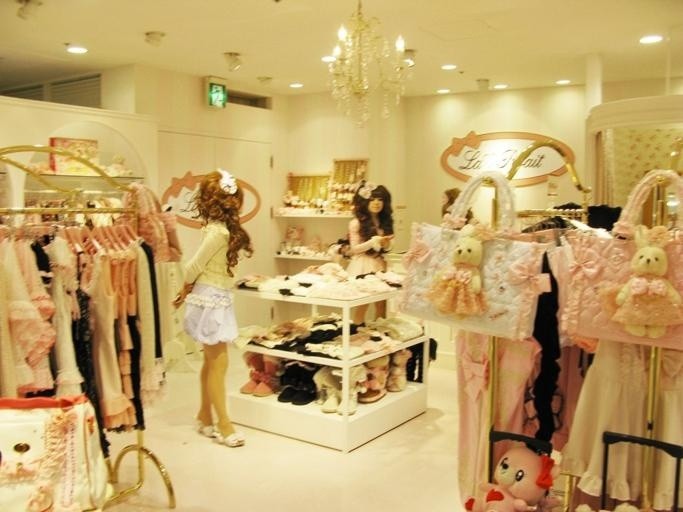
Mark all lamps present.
[227,54,242,72]
[399,48,416,69]
[17,1,38,21]
[322,0,408,127]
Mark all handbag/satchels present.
[557,168,683,351]
[0,397,108,512]
[397,172,550,341]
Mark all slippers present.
[194,419,217,438]
[214,424,245,447]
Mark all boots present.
[312,349,412,414]
[240,352,319,405]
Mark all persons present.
[172,169,254,448]
[441,188,474,224]
[348,179,394,324]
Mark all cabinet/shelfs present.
[270,206,354,261]
[225,287,429,453]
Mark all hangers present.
[1,208,177,266]
[518,206,587,234]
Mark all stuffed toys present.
[425,224,486,320]
[465,444,554,512]
[610,225,683,339]
